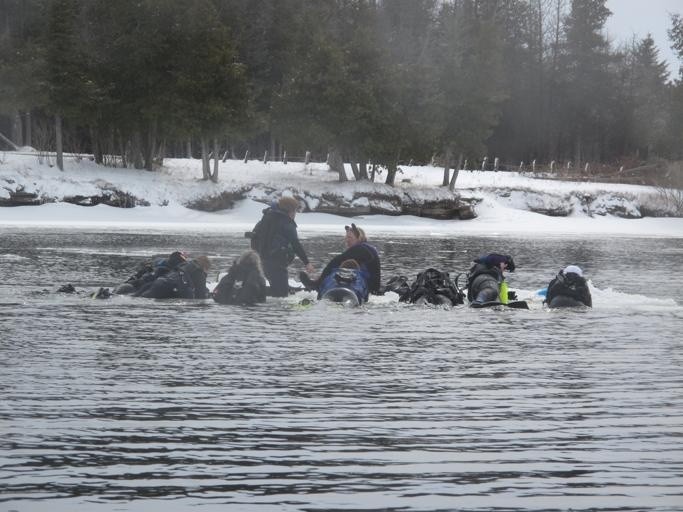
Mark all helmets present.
[564,266,582,276]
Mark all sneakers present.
[300,271,312,290]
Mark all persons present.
[297,222,380,294]
[317,259,370,310]
[545,265,591,308]
[468,251,515,306]
[132,255,211,300]
[116,250,187,297]
[251,195,312,298]
[211,249,265,305]
